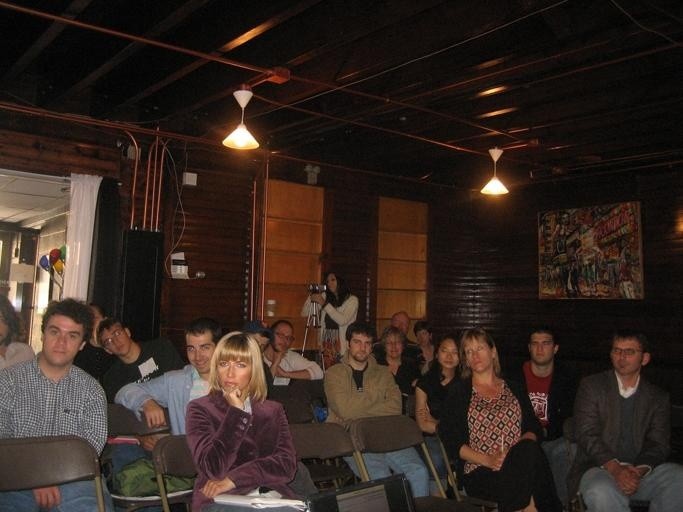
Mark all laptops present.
[311,473,414,512]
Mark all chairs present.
[562,417,585,512]
[1,435,105,511]
[104,403,195,512]
[268,379,498,511]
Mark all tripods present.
[300,300,322,361]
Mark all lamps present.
[479,147,513,197]
[221,86,263,151]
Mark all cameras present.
[308,284,327,291]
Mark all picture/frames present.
[533,202,647,304]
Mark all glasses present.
[274,331,295,342]
[102,327,125,348]
[611,347,644,355]
